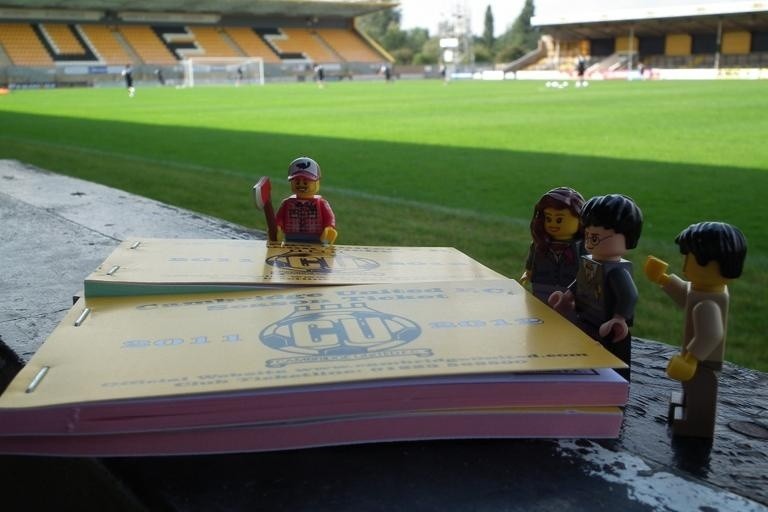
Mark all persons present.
[121,60,136,98]
[377,64,390,82]
[439,59,447,84]
[573,46,588,88]
[312,62,325,88]
[252,157,337,246]
[547,194,645,390]
[518,187,585,323]
[643,221,748,436]
[236,64,245,86]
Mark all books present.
[0,407,625,461]
[1,298,630,433]
[84,236,509,299]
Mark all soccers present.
[544,80,568,88]
[575,82,588,87]
